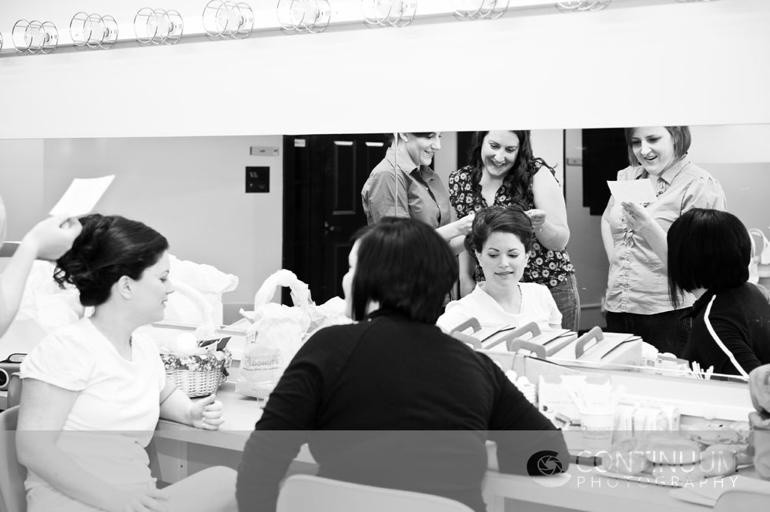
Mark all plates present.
[609,422,738,476]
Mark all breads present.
[602,430,752,483]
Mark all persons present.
[15,215,237,512]
[600,127,770,380]
[0,196,82,340]
[237,218,568,511]
[362,130,581,335]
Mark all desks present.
[0,326,769,511]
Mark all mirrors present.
[0,120,768,410]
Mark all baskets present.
[165,368,223,398]
